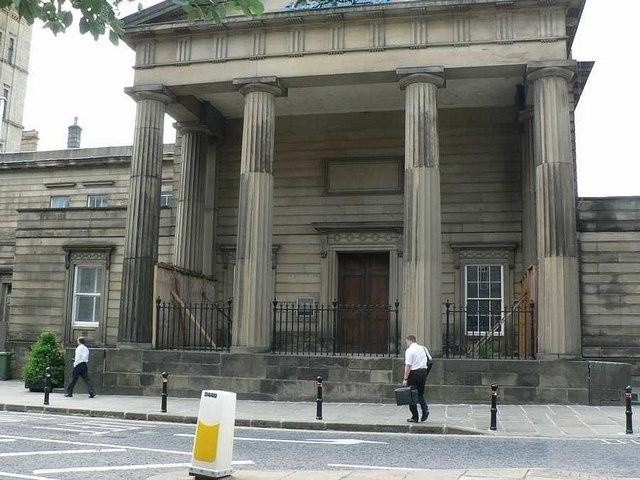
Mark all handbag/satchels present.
[394,383,420,405]
[426,355,432,376]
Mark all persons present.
[64,337,97,399]
[403,335,432,422]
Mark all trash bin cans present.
[0,351,15,380]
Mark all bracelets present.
[404,378,407,380]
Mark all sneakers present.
[405,410,430,424]
[64,391,97,399]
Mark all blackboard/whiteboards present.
[297,297,315,317]
